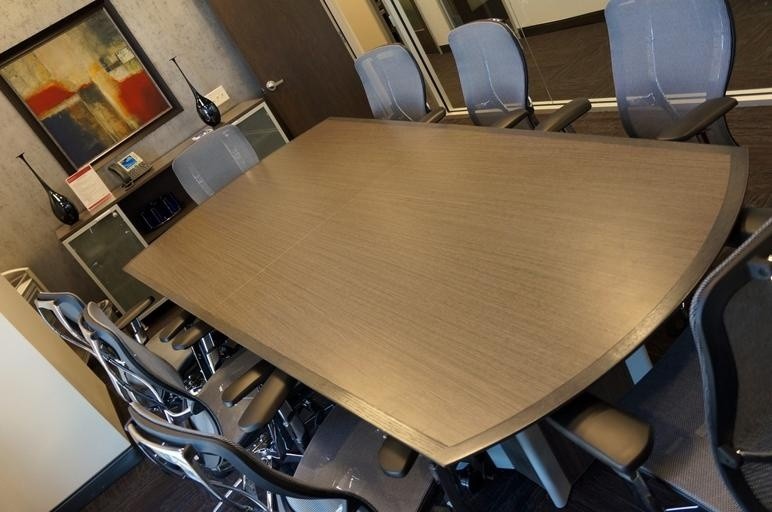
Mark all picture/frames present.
[0,0,184,177]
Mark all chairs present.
[171,124,261,206]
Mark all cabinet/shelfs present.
[54,95,292,322]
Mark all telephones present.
[105,150,153,187]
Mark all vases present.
[169,55,221,127]
[15,152,80,226]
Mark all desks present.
[122,114,750,510]
